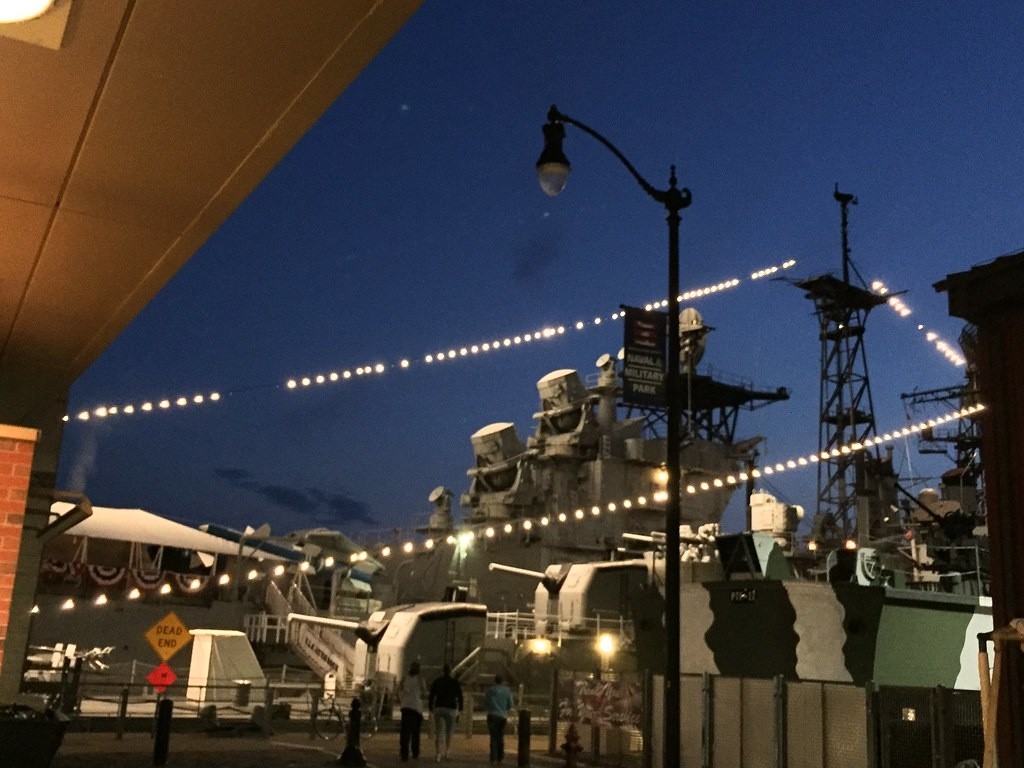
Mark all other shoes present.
[401,753,408,761]
[411,753,419,758]
[496,761,502,767]
[489,760,494,765]
[445,751,449,760]
[436,754,440,762]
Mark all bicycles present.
[313,693,377,742]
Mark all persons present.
[428,664,463,764]
[484,675,513,763]
[395,662,430,760]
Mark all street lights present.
[535,106,693,767]
[530,638,558,757]
[809,541,817,581]
